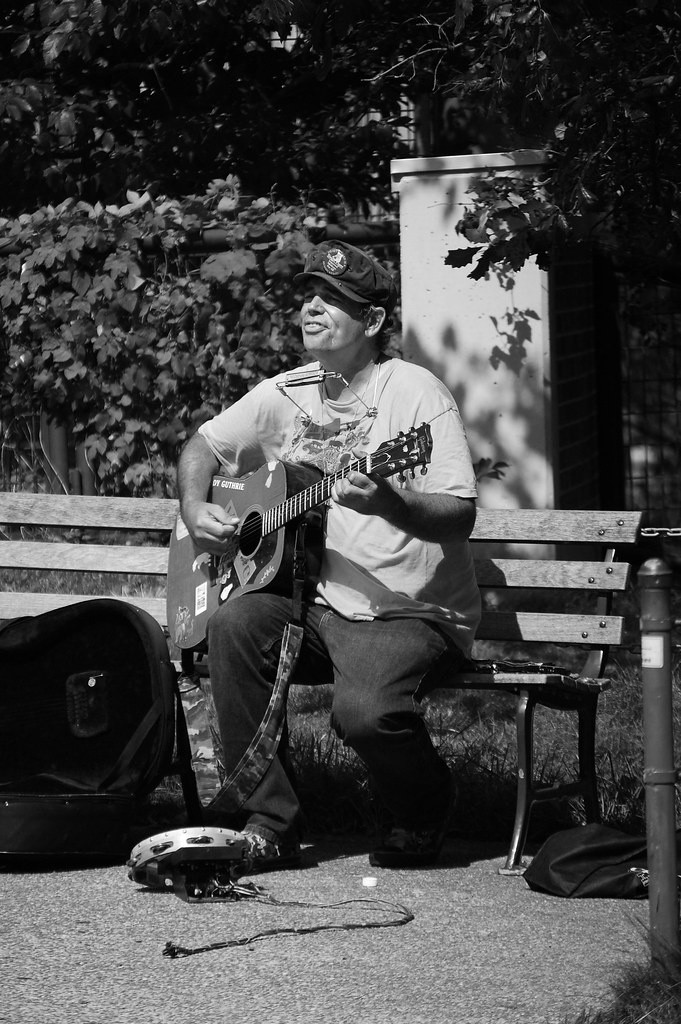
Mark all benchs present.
[0,491,642,874]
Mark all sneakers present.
[368,780,464,870]
[232,831,303,875]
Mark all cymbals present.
[128,826,253,891]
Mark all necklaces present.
[320,355,374,510]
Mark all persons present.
[172,246,486,869]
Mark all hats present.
[292,239,397,318]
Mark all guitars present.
[161,421,434,652]
